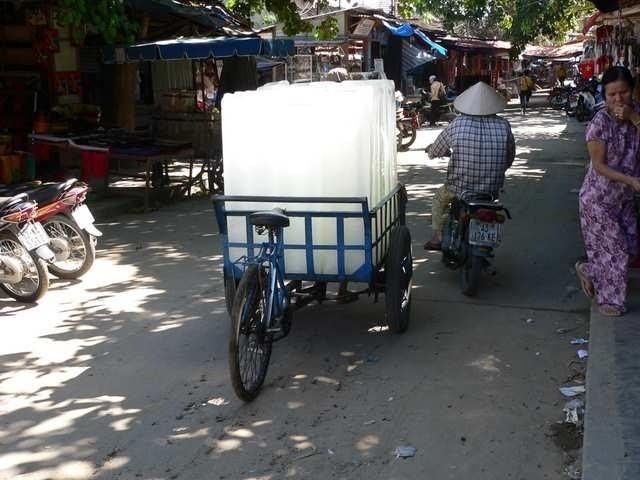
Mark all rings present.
[615,113,619,117]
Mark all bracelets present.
[632,120,640,128]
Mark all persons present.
[423,75,448,127]
[427,81,515,251]
[576,65,640,317]
[518,61,566,106]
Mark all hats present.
[454,80,507,115]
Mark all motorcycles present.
[0,174,104,280]
[423,140,509,298]
[0,192,55,303]
[396,84,464,153]
[547,75,603,122]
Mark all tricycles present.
[207,176,414,405]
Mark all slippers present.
[599,306,623,316]
[423,241,441,250]
[574,260,594,298]
